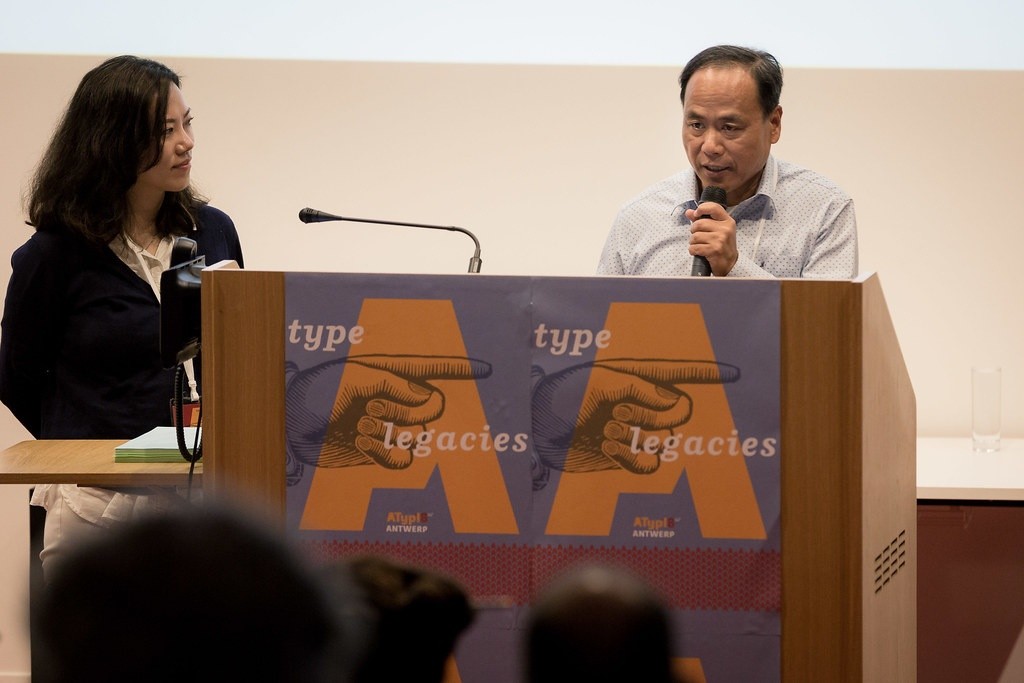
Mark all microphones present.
[300,208,481,273]
[690,184,727,277]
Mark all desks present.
[0,439,203,683]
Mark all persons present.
[596,45,860,281]
[26,488,695,683]
[0,55,246,581]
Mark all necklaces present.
[145,236,155,250]
